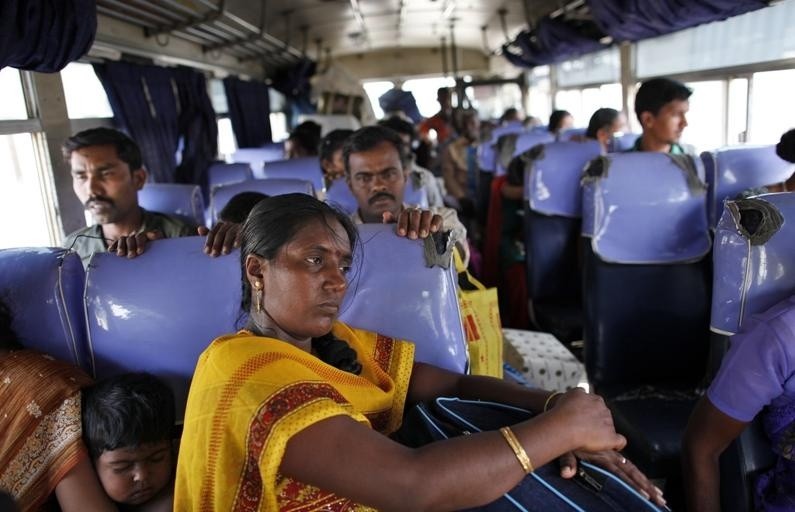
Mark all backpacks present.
[397,394,673,512]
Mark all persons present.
[548,80,694,153]
[736,128,795,200]
[682,294,795,511]
[62,111,469,274]
[2,192,665,511]
[378,80,547,237]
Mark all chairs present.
[474,122,794,509]
[1,142,473,435]
